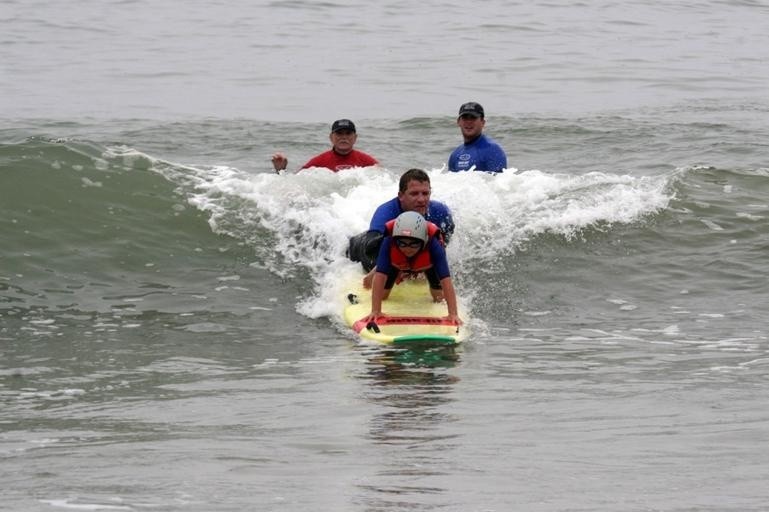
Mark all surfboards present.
[343,256,464,344]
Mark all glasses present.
[396,241,419,249]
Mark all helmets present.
[392,210,429,251]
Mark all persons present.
[363,209,464,326]
[447,101,506,174]
[367,170,456,248]
[269,119,380,174]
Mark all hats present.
[457,101,484,120]
[332,119,356,133]
